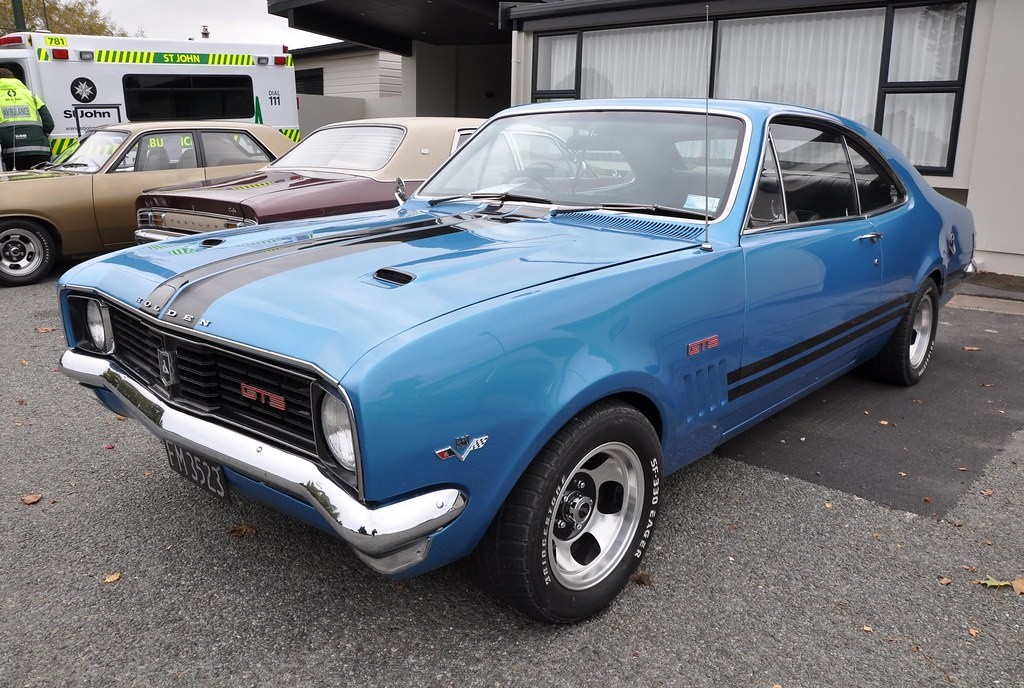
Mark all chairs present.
[144,147,169,171]
[175,149,197,169]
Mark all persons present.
[0,68,57,171]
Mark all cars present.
[0,121,301,289]
[134,116,627,246]
[57,98,978,626]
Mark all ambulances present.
[0,30,302,172]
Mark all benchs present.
[667,165,883,224]
